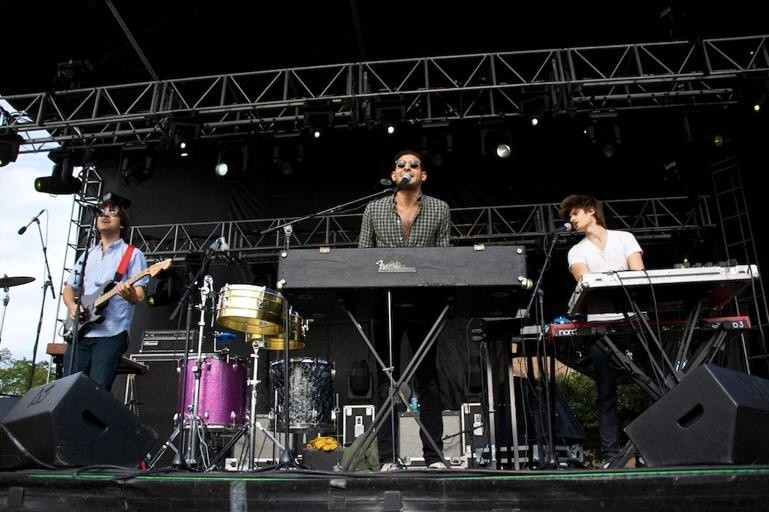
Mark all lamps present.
[0,70,768,194]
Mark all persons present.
[358,150,451,471]
[559,195,645,468]
[62,198,150,392]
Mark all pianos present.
[566,262,760,316]
[546,315,760,343]
[276,245,528,321]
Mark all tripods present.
[146,296,215,476]
[201,339,285,474]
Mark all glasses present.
[395,159,422,168]
[96,208,123,219]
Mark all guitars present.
[63,259,172,342]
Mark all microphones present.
[18,208,46,236]
[395,172,411,195]
[209,236,225,252]
[87,203,105,217]
[546,221,572,239]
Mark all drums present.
[173,352,247,435]
[244,307,306,350]
[216,284,282,336]
[270,358,336,431]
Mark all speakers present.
[622,362,768,467]
[0,370,158,472]
